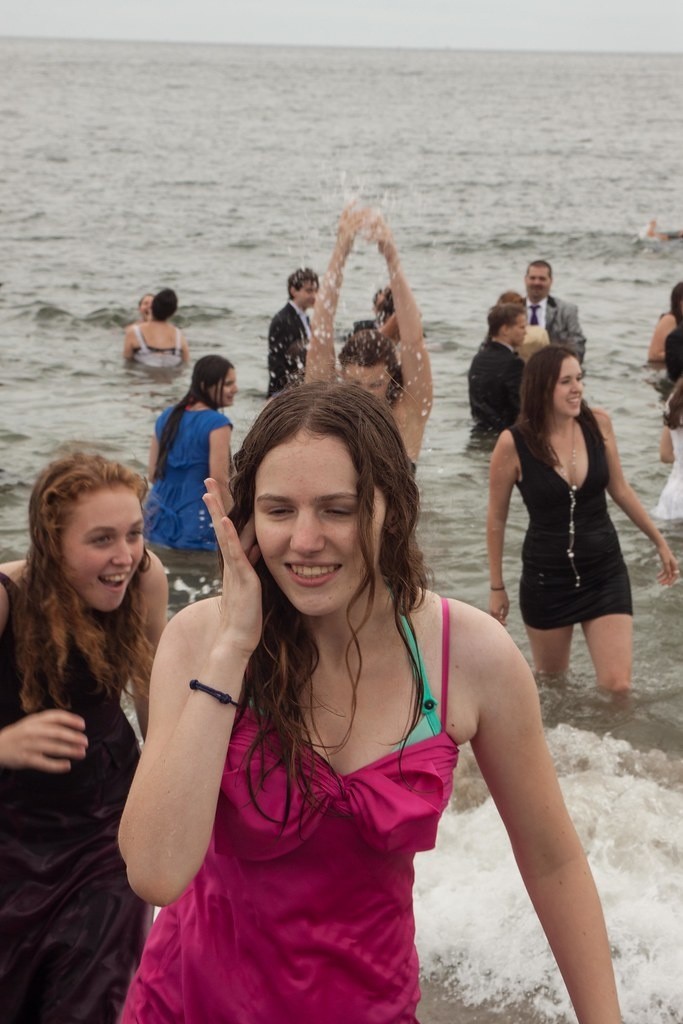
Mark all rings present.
[671,569,680,574]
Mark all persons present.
[117,382,625,1024]
[266,199,434,476]
[123,289,189,367]
[142,354,238,550]
[647,283,683,519]
[466,260,588,451]
[487,345,680,695]
[0,450,169,1024]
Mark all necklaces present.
[547,417,581,589]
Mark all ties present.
[305,316,312,331]
[529,303,540,323]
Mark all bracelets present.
[189,679,240,708]
[490,585,505,591]
